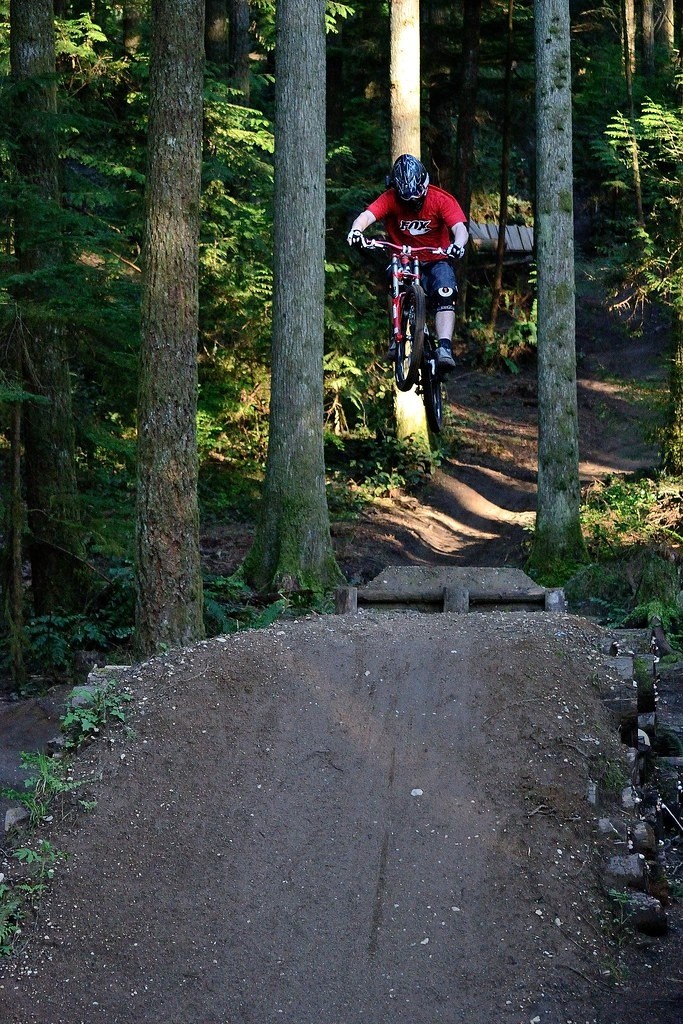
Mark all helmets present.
[392,153,427,203]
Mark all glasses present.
[400,172,429,200]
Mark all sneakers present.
[435,346,456,368]
[387,341,396,357]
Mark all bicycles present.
[361,238,447,434]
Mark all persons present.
[347,154,469,373]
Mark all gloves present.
[347,229,363,248]
[445,243,465,259]
